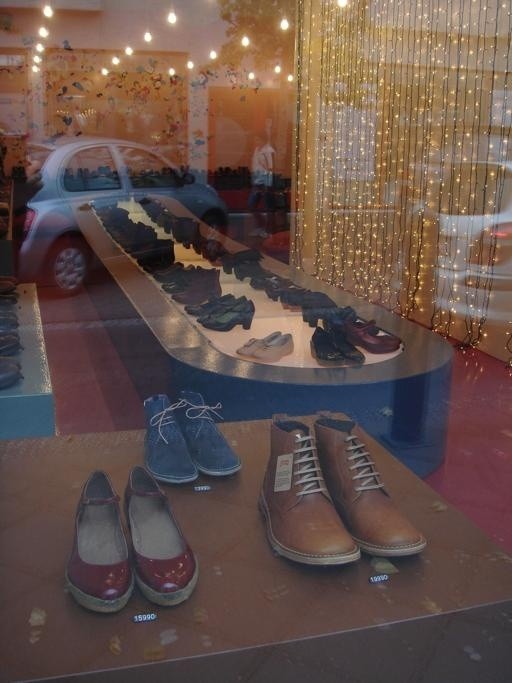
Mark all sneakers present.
[248,228,272,239]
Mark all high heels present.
[91,192,402,367]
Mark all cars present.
[389,161,512,323]
[15,133,231,298]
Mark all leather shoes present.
[65,464,199,614]
[257,412,428,565]
[64,165,252,191]
[143,390,242,483]
[0,275,21,392]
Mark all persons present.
[244,125,278,240]
